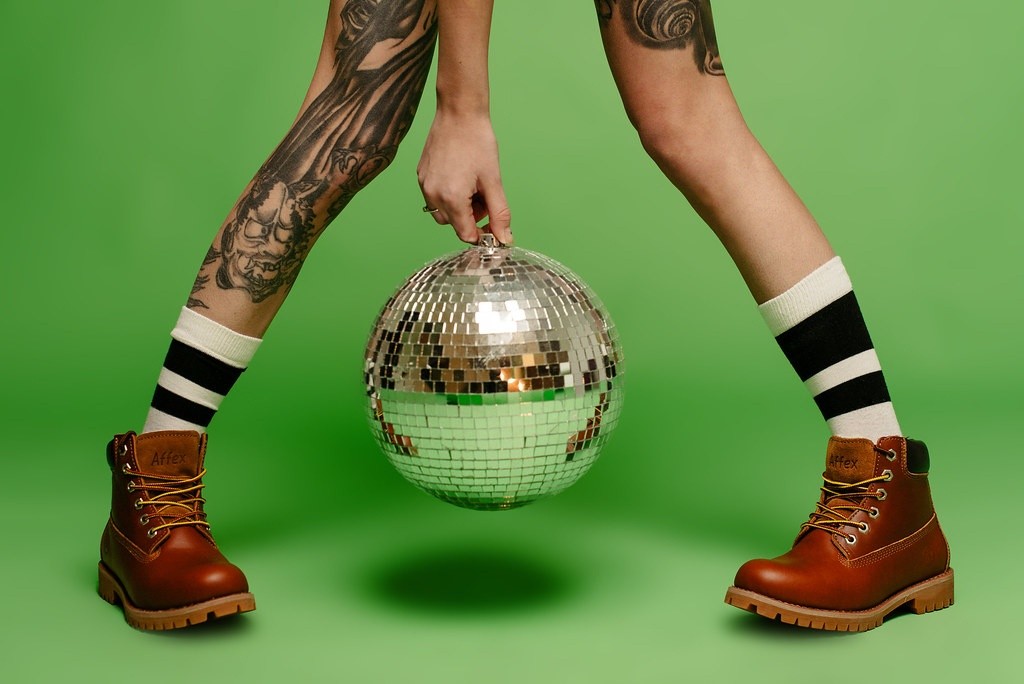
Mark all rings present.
[422,206,438,215]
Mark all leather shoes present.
[96,431,256,628]
[724,434,955,632]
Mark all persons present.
[94,0,955,634]
